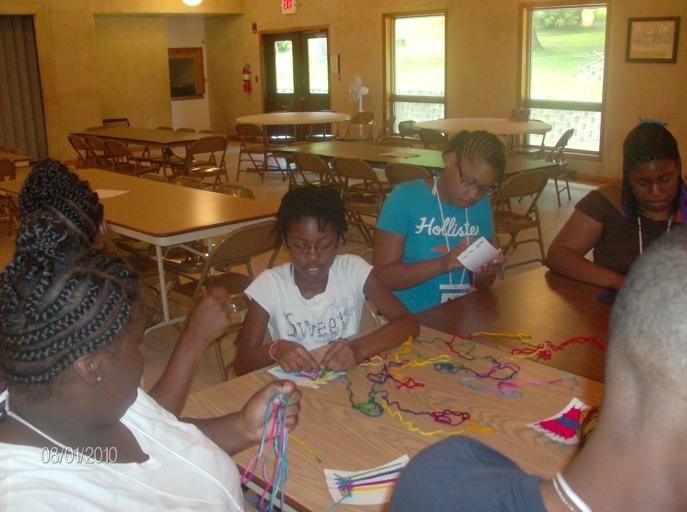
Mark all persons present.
[1,158,303,512]
[546,123,687,291]
[234,190,420,372]
[387,226,687,511]
[371,130,506,315]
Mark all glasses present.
[283,234,346,256]
[457,158,501,197]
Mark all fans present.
[345,74,370,113]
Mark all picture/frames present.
[626,15,680,64]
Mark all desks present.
[179,267,617,512]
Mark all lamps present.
[182,0,202,6]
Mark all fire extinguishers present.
[243,64,251,92]
